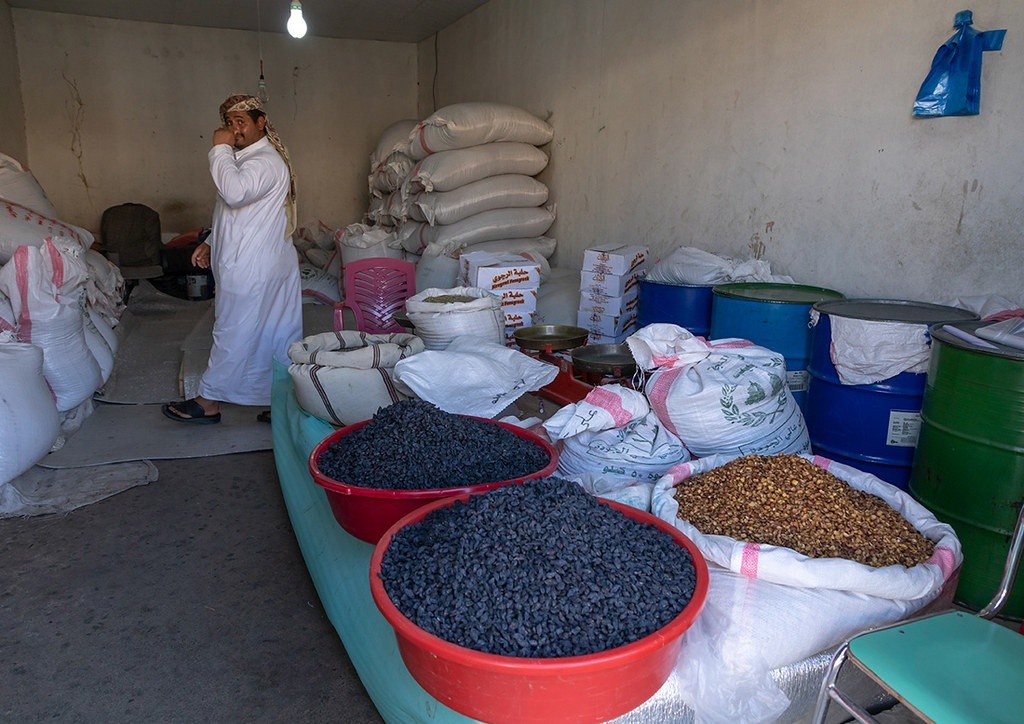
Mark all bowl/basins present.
[369,490,710,723]
[309,415,559,545]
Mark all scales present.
[513,324,637,407]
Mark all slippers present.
[258,410,273,423]
[162,398,222,423]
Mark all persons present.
[162,95,302,424]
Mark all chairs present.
[100,204,176,297]
[813,508,1024,724]
[332,257,417,331]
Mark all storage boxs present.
[455,250,542,350]
[577,243,652,346]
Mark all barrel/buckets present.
[632,273,712,341]
[801,297,981,490]
[710,282,849,409]
[906,319,1024,623]
